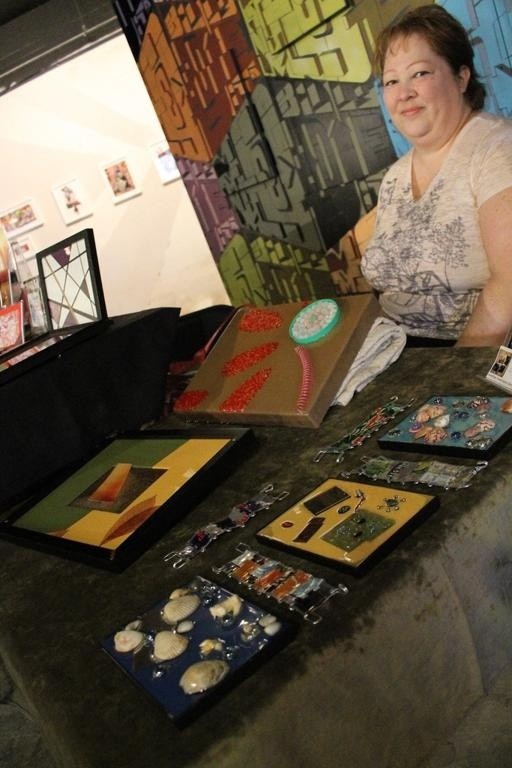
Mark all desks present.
[0,345,511,767]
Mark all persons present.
[358,2,512,350]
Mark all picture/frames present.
[0,426,260,573]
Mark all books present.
[0,300,25,348]
